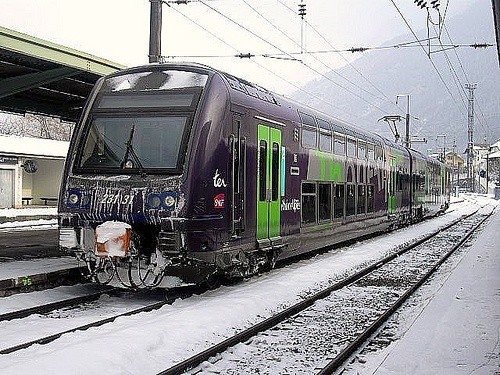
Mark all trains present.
[56,61,455,292]
